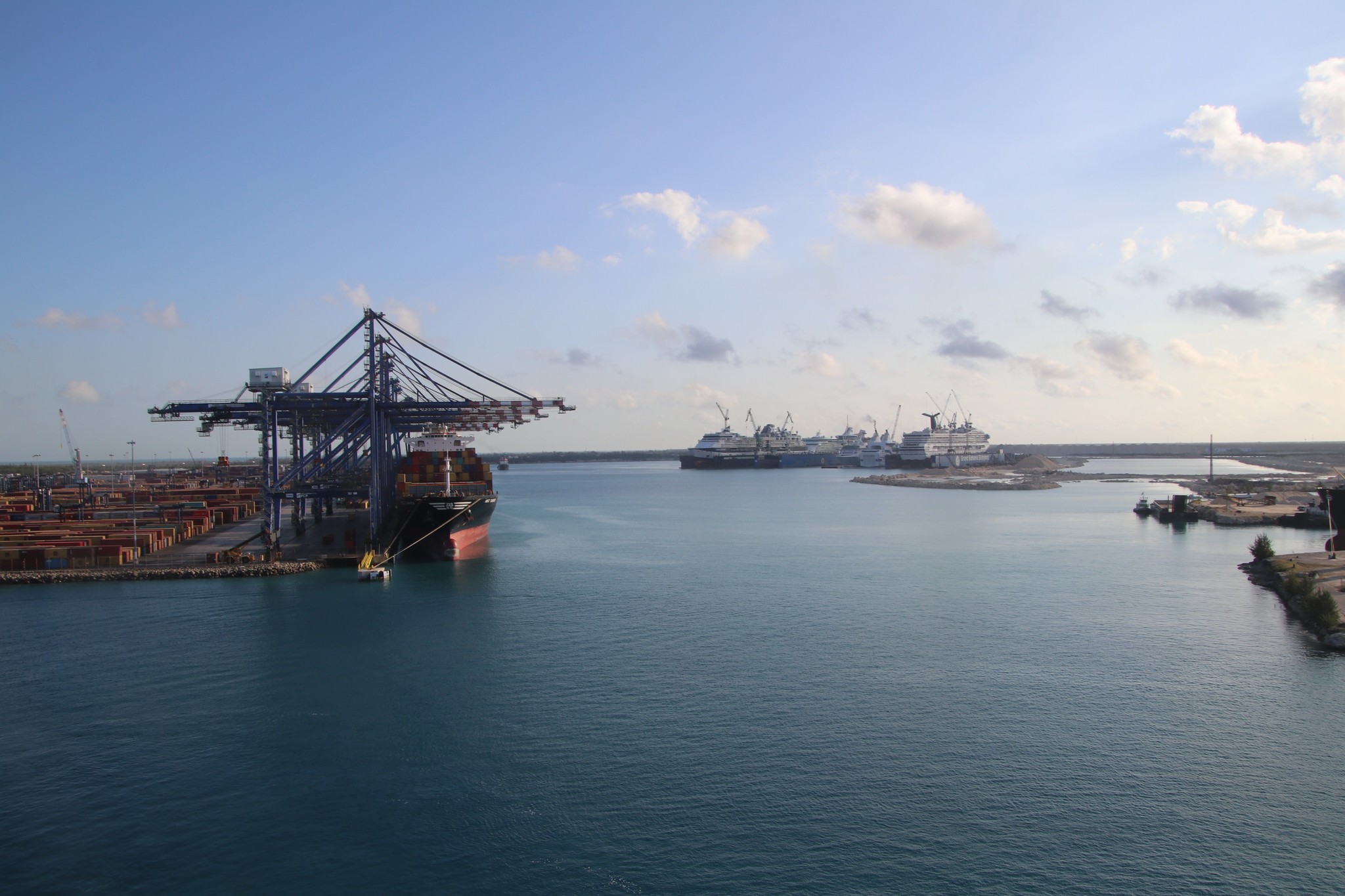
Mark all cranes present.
[148,307,580,576]
[59,408,88,485]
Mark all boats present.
[677,400,862,470]
[897,386,990,465]
[835,414,866,467]
[393,421,499,556]
[859,405,906,467]
[498,454,509,470]
[357,566,392,581]
[1133,491,1152,513]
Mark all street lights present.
[168,451,172,483]
[85,455,89,479]
[127,440,139,565]
[109,454,114,498]
[32,454,41,489]
[200,451,204,477]
